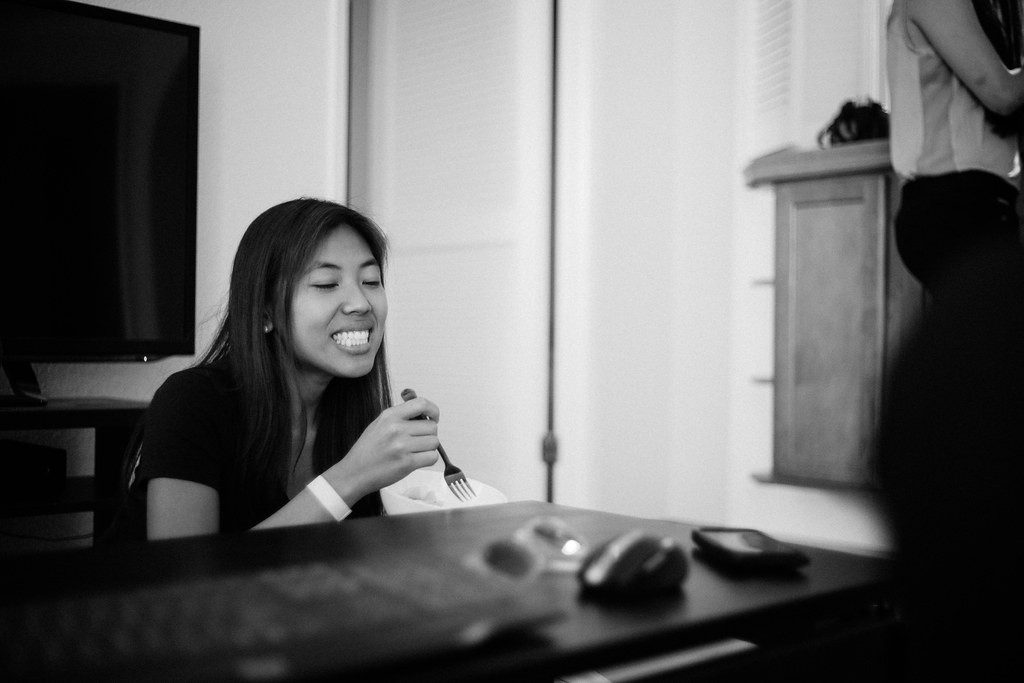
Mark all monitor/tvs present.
[0,0,200,403]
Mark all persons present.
[884,0,1023,297]
[123,200,439,541]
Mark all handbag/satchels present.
[818,101,889,145]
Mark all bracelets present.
[306,476,353,522]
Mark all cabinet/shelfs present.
[745,142,944,489]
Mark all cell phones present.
[691,527,806,570]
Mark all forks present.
[400,388,477,502]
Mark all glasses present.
[469,516,588,587]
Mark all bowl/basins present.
[380,469,507,516]
